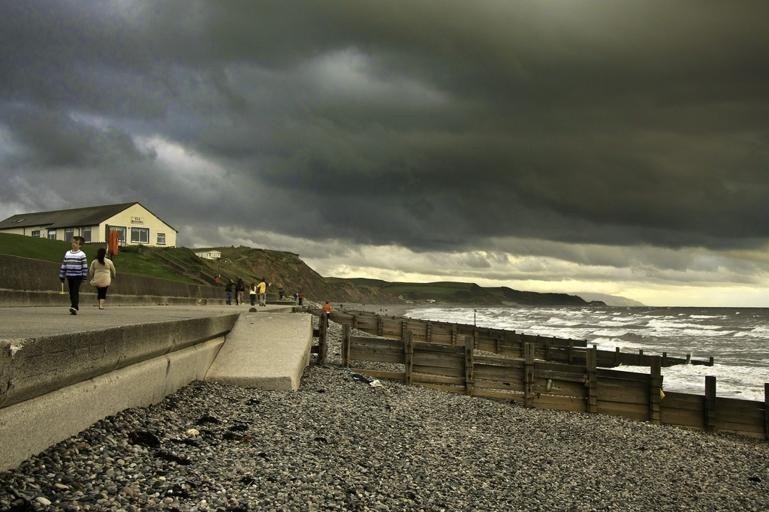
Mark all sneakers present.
[70,308,78,314]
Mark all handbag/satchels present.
[90,280,95,287]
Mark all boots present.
[99,299,105,310]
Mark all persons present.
[59,237,88,315]
[89,248,116,310]
[279,287,304,305]
[225,277,272,307]
[322,301,333,330]
